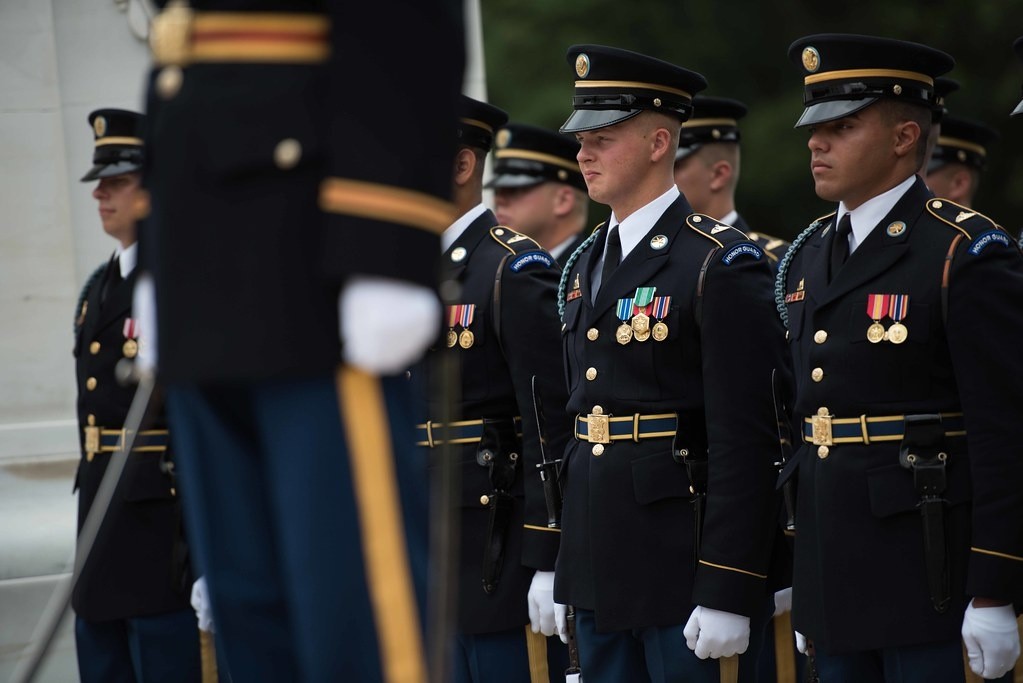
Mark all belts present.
[570,413,675,444]
[801,412,968,446]
[413,417,524,448]
[139,3,331,64]
[81,424,172,453]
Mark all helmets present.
[459,95,508,152]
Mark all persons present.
[774,34,1023,683]
[927,116,1000,225]
[408,90,568,683]
[672,94,794,615]
[133,0,468,682]
[553,45,775,683]
[480,119,589,269]
[72,108,213,683]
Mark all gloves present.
[528,569,570,644]
[961,596,1019,682]
[795,632,811,661]
[337,274,443,376]
[684,604,751,659]
[773,588,794,618]
[189,575,215,635]
[130,273,159,376]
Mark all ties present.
[602,224,622,287]
[828,215,852,285]
[105,256,121,298]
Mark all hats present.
[676,96,747,163]
[484,122,588,194]
[558,43,709,133]
[927,118,993,173]
[787,35,955,130]
[80,109,151,181]
[929,79,960,121]
